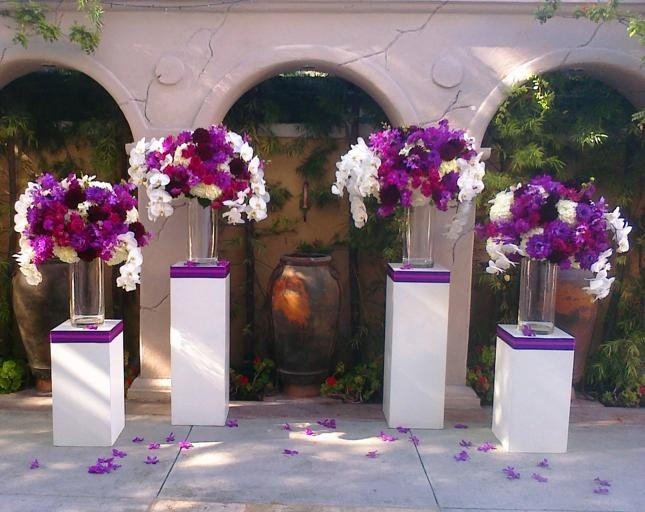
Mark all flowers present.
[15,171,152,292]
[485,175,632,303]
[126,122,271,226]
[329,115,485,244]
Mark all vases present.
[519,258,556,334]
[269,252,354,385]
[557,283,600,387]
[10,260,72,379]
[68,254,104,329]
